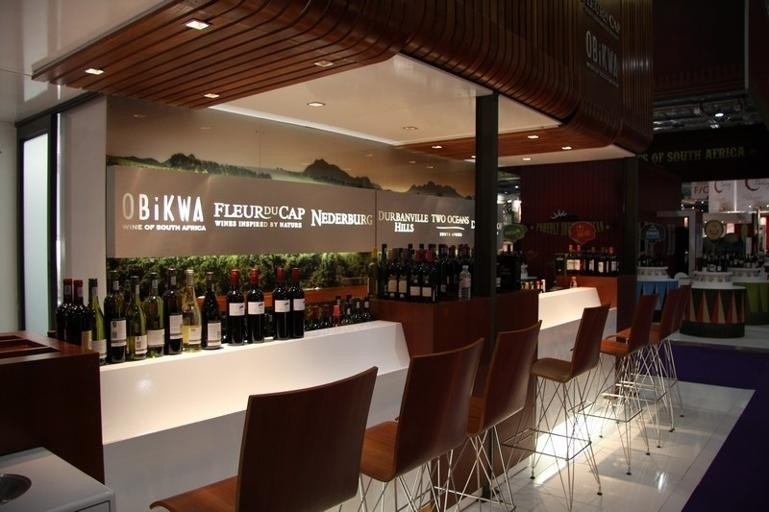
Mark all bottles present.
[308,294,374,333]
[520,278,554,294]
[571,276,577,290]
[54,266,305,365]
[368,242,527,302]
[637,249,765,274]
[566,243,619,276]
